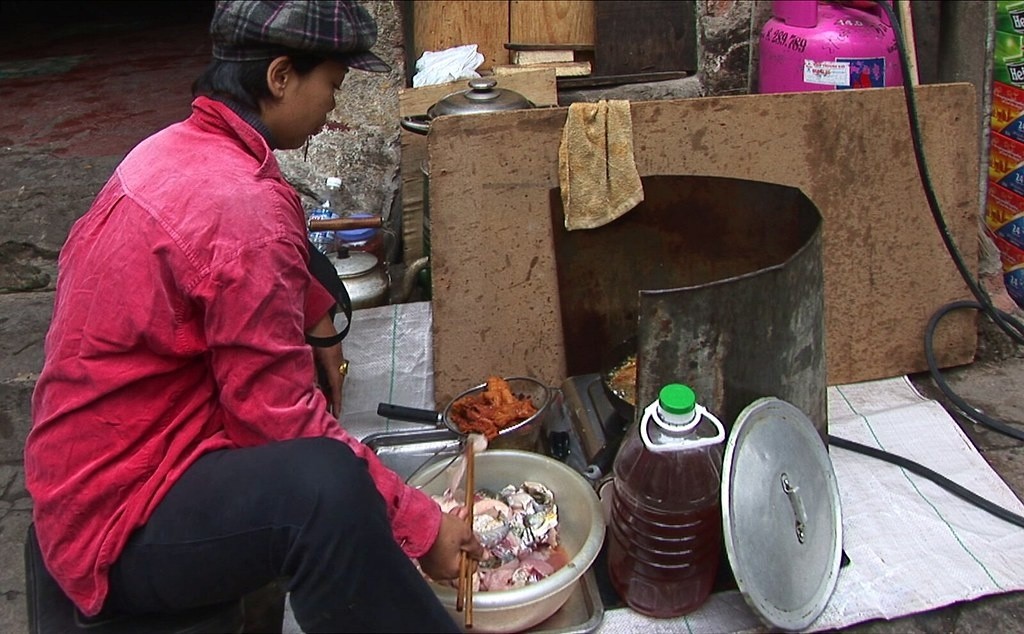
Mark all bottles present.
[307,176,345,255]
[608,382,727,620]
[336,212,385,267]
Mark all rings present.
[338,359,350,375]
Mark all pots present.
[603,330,639,423]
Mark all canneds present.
[336,214,384,267]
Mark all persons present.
[25,0,486,634]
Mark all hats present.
[210,0,392,73]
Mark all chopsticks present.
[456,438,474,628]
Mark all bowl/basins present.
[399,449,607,634]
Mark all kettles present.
[306,215,430,313]
